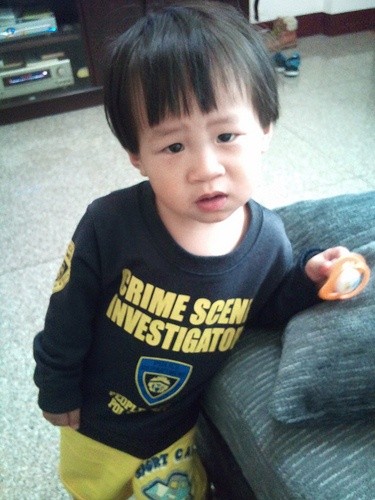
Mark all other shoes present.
[262,15,298,52]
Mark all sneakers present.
[270,52,301,76]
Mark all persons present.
[30,1,361,500]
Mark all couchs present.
[189,192,372,500]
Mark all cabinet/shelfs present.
[0,0,257,122]
